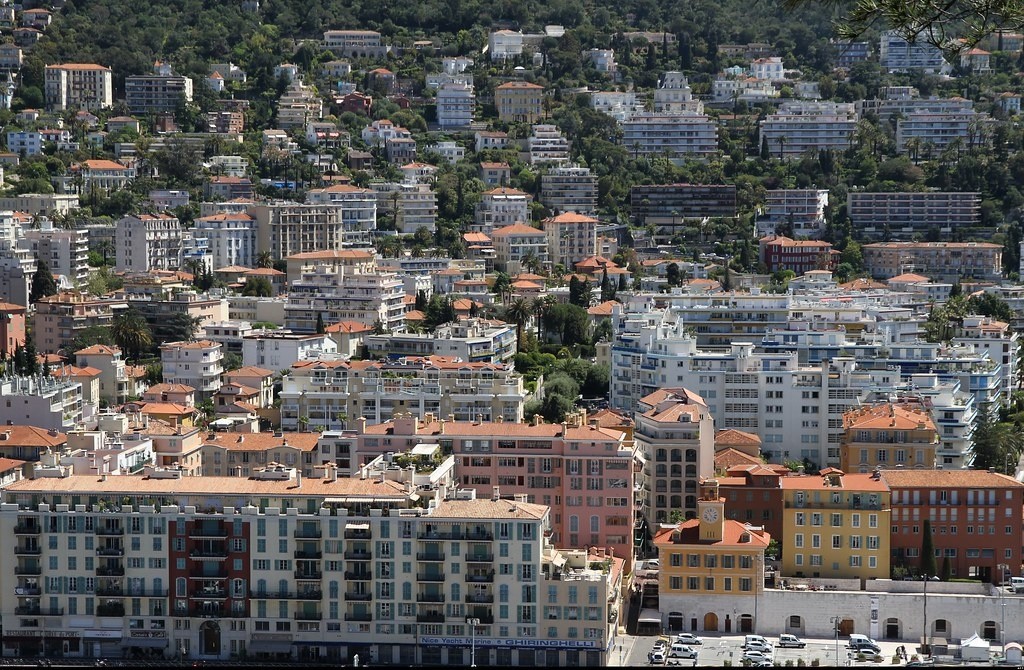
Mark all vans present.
[1008,577,1024,590]
[745,635,772,646]
[848,633,885,662]
[779,633,806,649]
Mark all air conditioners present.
[186,235,190,239]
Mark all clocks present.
[702,507,719,524]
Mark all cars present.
[752,662,774,667]
[742,651,773,662]
[745,642,771,652]
[649,635,698,665]
[641,560,659,570]
[677,633,703,646]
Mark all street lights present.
[922,574,941,653]
